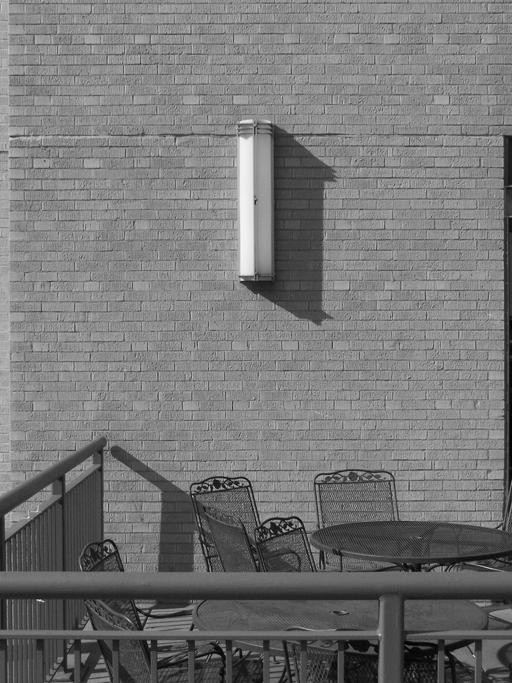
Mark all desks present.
[311,517,512,572]
[194,597,490,681]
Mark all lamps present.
[236,117,279,283]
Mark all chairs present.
[77,538,226,680]
